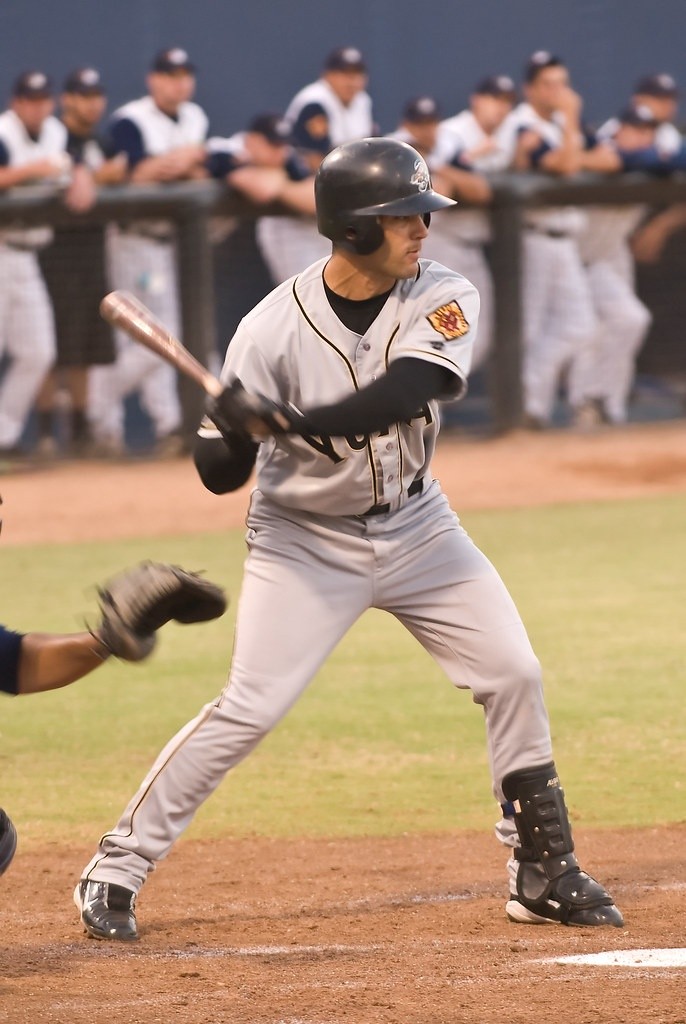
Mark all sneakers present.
[73,878,139,941]
[505,870,626,928]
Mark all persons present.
[206,114,316,246]
[72,137,625,940]
[437,76,518,373]
[581,72,686,423]
[36,67,128,459]
[0,70,96,464]
[390,97,489,202]
[493,49,603,428]
[84,48,251,459]
[257,44,374,282]
[0,489,228,879]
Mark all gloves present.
[205,377,296,447]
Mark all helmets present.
[526,51,561,80]
[17,70,53,99]
[64,68,103,96]
[153,47,194,75]
[313,138,458,256]
[470,73,516,100]
[406,95,438,121]
[325,46,364,71]
[636,73,678,99]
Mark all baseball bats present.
[99,287,268,433]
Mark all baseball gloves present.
[96,560,226,661]
